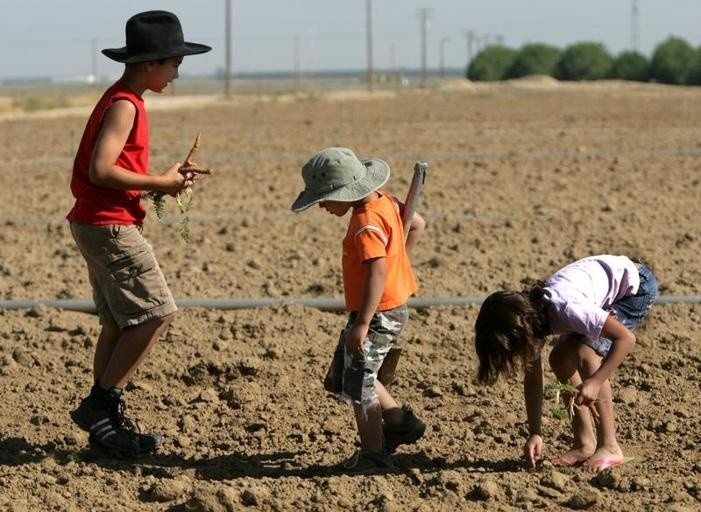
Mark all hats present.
[101,11,212,64]
[291,147,390,212]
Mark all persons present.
[66,10,211,459]
[291,147,427,473]
[475,253,658,475]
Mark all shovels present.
[324,161,426,393]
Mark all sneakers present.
[326,404,426,475]
[71,378,162,460]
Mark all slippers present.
[552,457,634,472]
